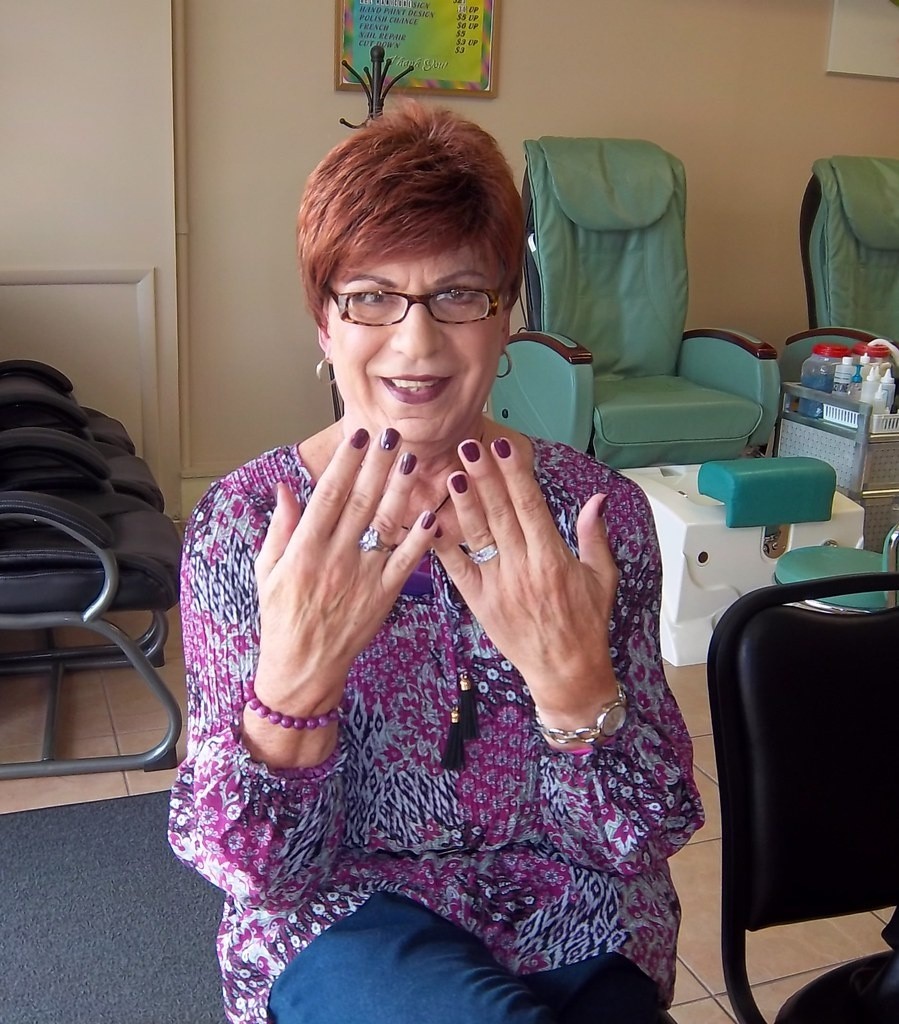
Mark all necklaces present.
[398,433,485,530]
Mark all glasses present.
[326,279,505,327]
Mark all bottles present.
[797,343,851,417]
[881,369,895,414]
[875,366,881,383]
[853,342,896,378]
[873,384,886,413]
[862,367,879,403]
[859,353,870,367]
[832,357,854,397]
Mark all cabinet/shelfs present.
[780,381,899,555]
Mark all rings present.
[469,544,498,563]
[358,526,395,552]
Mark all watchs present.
[535,682,627,743]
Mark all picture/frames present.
[334,0,503,99]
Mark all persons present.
[168,106,706,1024]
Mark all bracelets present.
[243,676,338,730]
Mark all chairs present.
[777,152,899,404]
[705,569,899,1023]
[480,136,782,468]
[0,357,183,773]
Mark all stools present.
[776,520,899,615]
